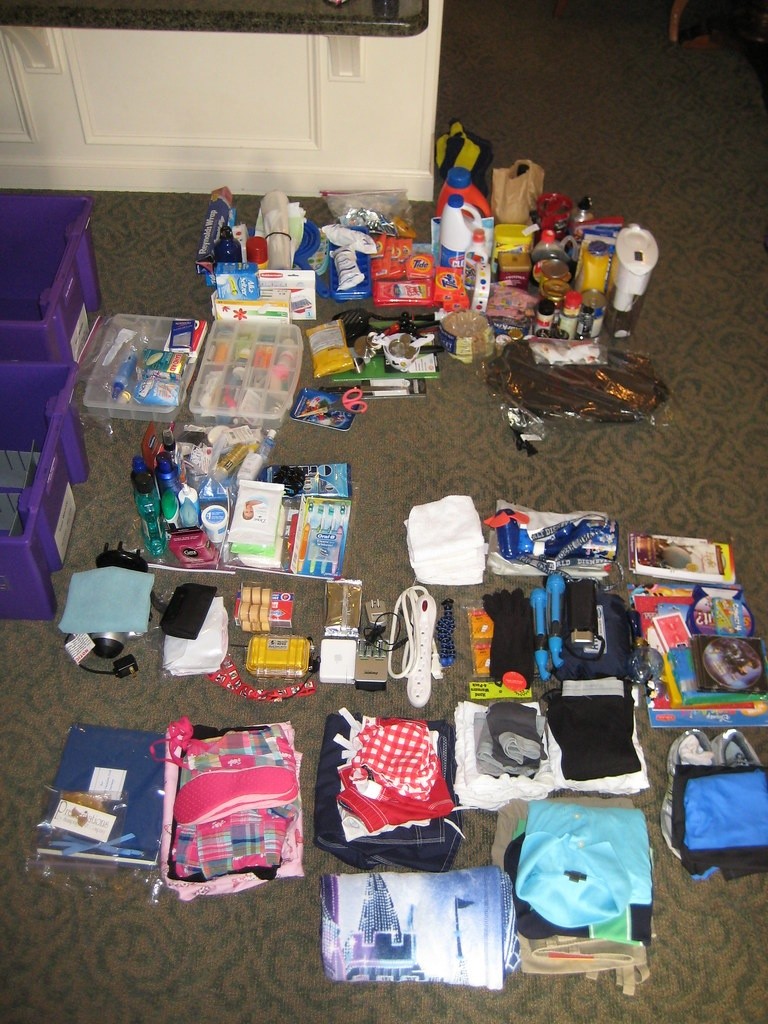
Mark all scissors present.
[293,387,368,418]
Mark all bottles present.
[215,226,242,263]
[535,229,578,263]
[441,195,480,267]
[437,167,491,217]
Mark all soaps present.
[371,236,471,311]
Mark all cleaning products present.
[436,166,492,292]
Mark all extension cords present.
[405,594,437,708]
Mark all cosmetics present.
[126,418,287,571]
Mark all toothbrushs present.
[297,500,346,574]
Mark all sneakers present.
[660,729,763,860]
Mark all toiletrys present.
[130,419,201,558]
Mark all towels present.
[402,493,486,586]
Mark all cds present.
[693,596,762,689]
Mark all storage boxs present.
[439,312,495,364]
[214,289,293,325]
[0,194,102,365]
[245,633,310,678]
[234,591,295,629]
[82,313,197,423]
[215,262,260,300]
[490,224,533,273]
[497,251,532,290]
[189,319,304,429]
[0,362,90,621]
[168,526,220,568]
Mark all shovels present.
[331,307,436,341]
